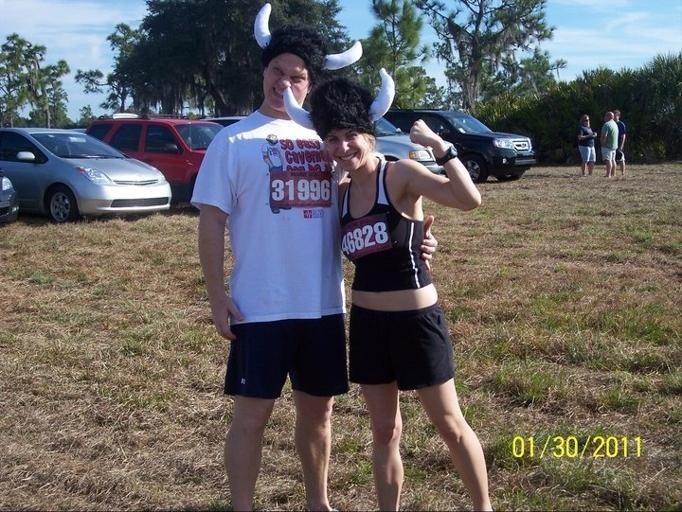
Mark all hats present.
[261,23,329,86]
[310,78,376,142]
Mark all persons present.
[284,69,491,511]
[191,24,439,511]
[578,110,626,178]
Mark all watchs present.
[436,145,458,167]
